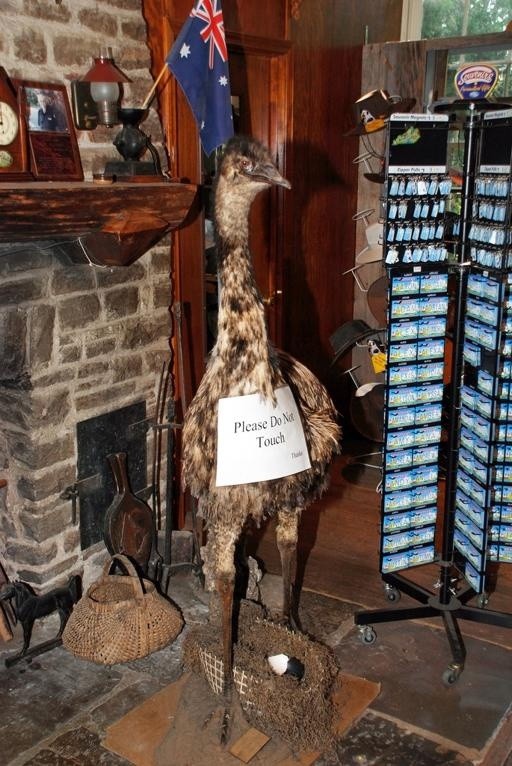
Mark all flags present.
[163,0,237,159]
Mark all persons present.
[35,93,63,130]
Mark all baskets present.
[61,554,186,666]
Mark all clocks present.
[0,66,35,182]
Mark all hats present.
[328,319,387,368]
[341,88,416,138]
[355,221,384,264]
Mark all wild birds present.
[179,135,345,752]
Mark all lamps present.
[71,46,128,130]
